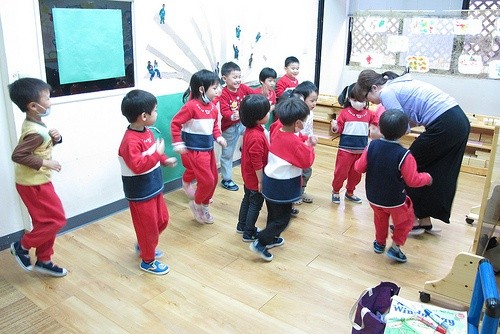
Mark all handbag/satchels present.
[349,280,396,334]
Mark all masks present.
[350,99,366,111]
[34,102,51,117]
[295,120,305,132]
[202,87,210,103]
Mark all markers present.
[424,308,449,329]
[418,315,446,334]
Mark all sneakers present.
[332,193,340,204]
[291,207,300,217]
[242,229,263,242]
[140,259,169,275]
[190,199,205,223]
[34,260,67,277]
[373,239,386,253]
[11,240,33,272]
[202,206,214,224]
[345,193,362,203]
[221,179,239,191]
[265,237,284,249]
[249,239,273,261]
[136,244,163,257]
[183,182,195,197]
[386,245,408,262]
[236,226,259,234]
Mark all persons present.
[236,94,270,243]
[249,98,314,262]
[353,69,471,235]
[9,77,68,277]
[253,67,277,112]
[274,56,300,104]
[220,62,267,191]
[117,90,179,275]
[273,80,319,217]
[354,109,433,262]
[171,69,228,225]
[331,83,382,204]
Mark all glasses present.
[364,86,371,102]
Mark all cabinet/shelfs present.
[312,95,499,180]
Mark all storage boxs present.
[460,133,493,168]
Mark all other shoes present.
[302,193,313,203]
[389,224,425,235]
[292,198,302,204]
[416,220,442,234]
